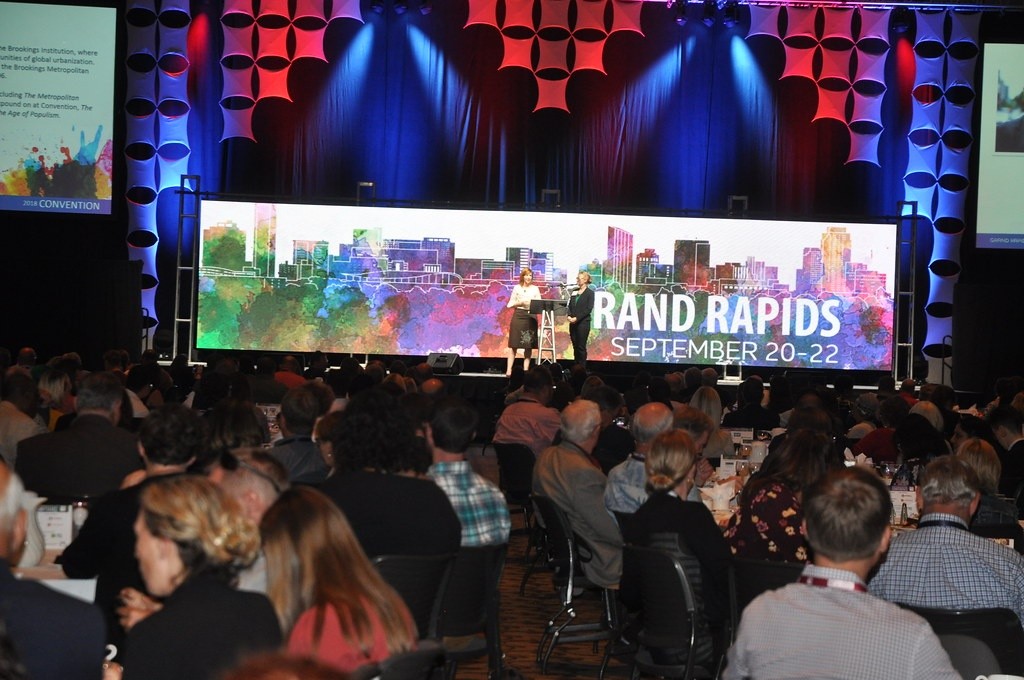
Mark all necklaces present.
[523,284,527,294]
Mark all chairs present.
[374,441,1024,680]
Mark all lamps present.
[891,7,910,33]
[369,0,433,16]
[672,0,741,29]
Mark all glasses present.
[220,447,281,494]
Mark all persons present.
[566,271,594,367]
[0,347,1024,680]
[507,268,542,376]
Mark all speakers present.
[57,259,144,372]
[427,353,464,375]
[786,371,854,391]
[952,286,1024,393]
